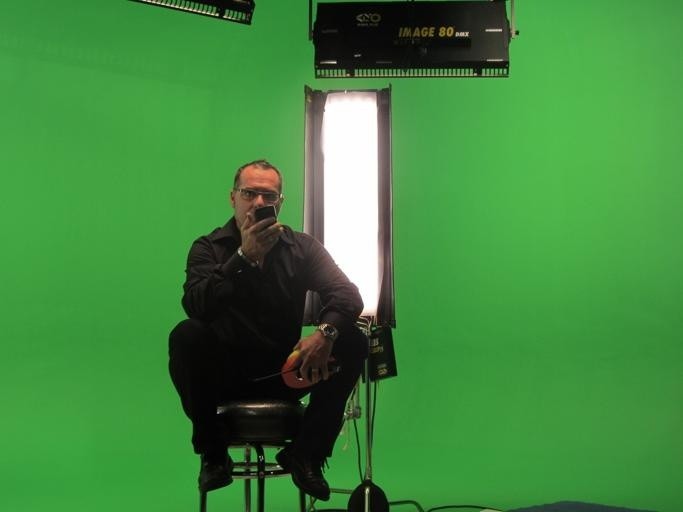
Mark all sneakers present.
[197,447,234,493]
[274,444,331,502]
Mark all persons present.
[163,154,372,503]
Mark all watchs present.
[314,320,340,342]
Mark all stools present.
[197,401,306,512]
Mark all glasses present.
[232,187,283,202]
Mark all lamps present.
[304,83,396,512]
[138,0,256,24]
[308,0,518,81]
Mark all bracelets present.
[235,245,262,268]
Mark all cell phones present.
[255,206,276,221]
